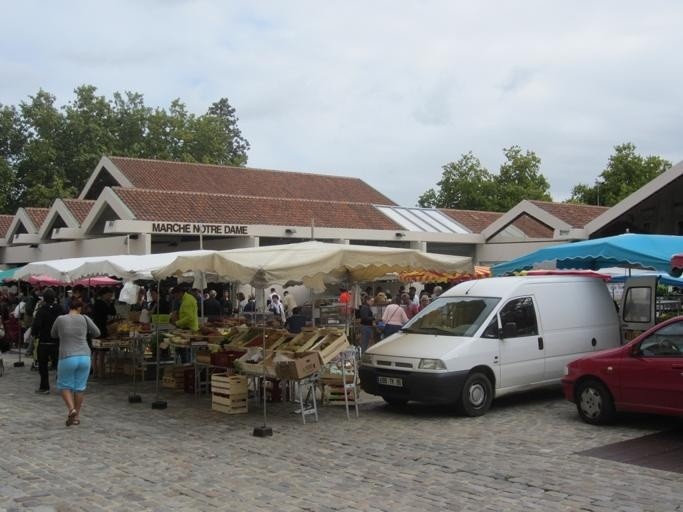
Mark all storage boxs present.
[162,328,350,416]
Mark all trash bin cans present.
[184,369,194,393]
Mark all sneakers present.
[36,390,49,394]
[65,409,80,426]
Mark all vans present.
[358,273,659,419]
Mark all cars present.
[559,316,682,427]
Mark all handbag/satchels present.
[82,314,92,347]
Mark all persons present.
[49,299,101,426]
[234,287,298,322]
[0,279,233,394]
[338,285,442,338]
